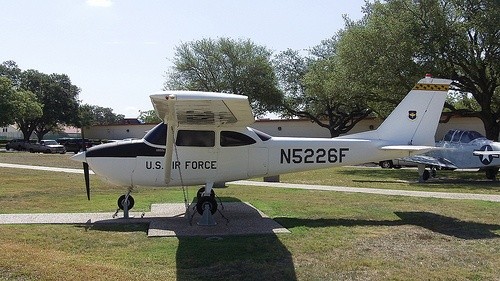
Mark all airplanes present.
[69,73,453,226]
[399,127,500,183]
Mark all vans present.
[57,138,89,153]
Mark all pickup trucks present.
[6,138,28,151]
[29,140,66,154]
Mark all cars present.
[374,155,440,170]
